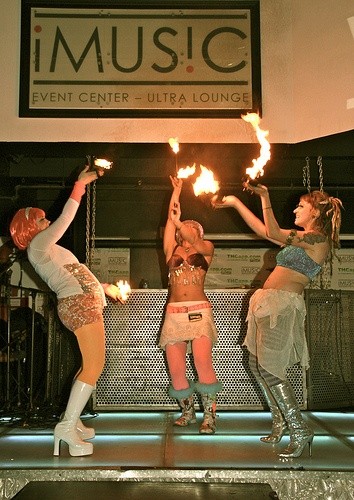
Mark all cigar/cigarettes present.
[242,179,250,191]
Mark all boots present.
[194,379,222,434]
[269,379,315,458]
[256,378,289,443]
[169,385,197,427]
[54,379,95,457]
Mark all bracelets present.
[262,206,273,211]
[177,223,184,231]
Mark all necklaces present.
[183,245,193,253]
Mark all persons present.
[9,166,126,457]
[211,182,342,458]
[158,175,221,435]
